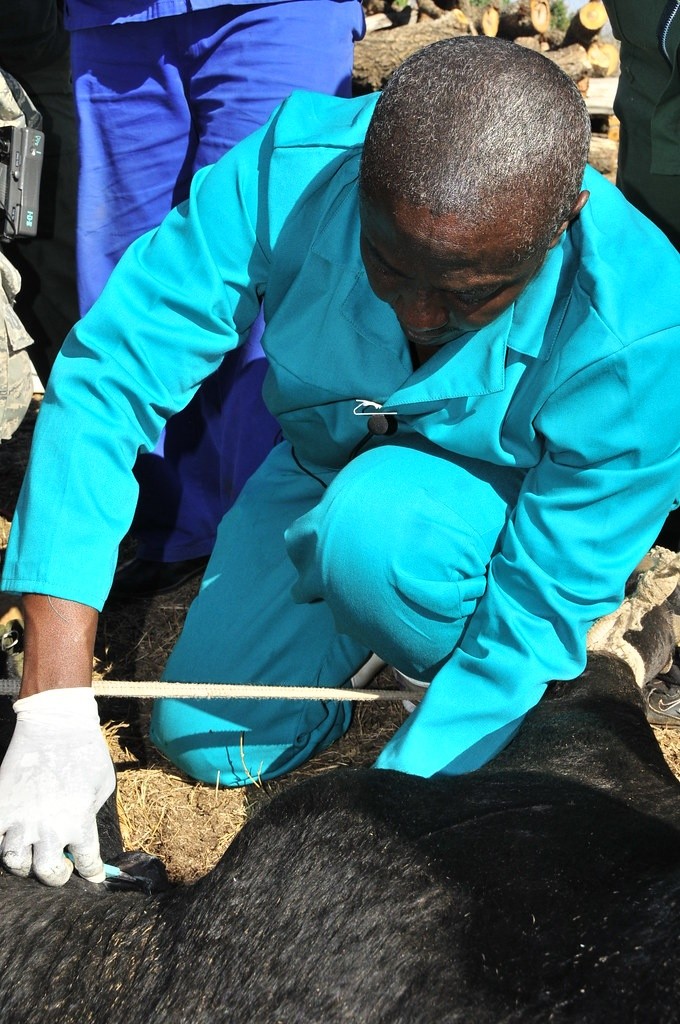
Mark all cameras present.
[0,124,45,242]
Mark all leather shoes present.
[108,556,210,596]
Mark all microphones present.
[368,415,398,437]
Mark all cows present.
[2,551,680,1024]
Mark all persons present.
[0,35,680,887]
[65,0,367,597]
[0,0,81,484]
[601,0,680,723]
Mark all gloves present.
[0,687,115,887]
